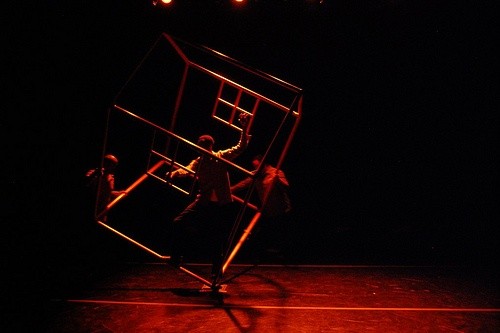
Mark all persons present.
[164,111,252,274]
[229,153,294,276]
[66,153,127,298]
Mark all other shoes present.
[211,264,223,278]
